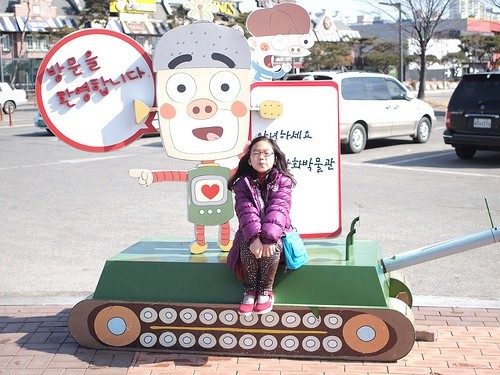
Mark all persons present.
[226,136,297,316]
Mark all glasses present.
[252,150,275,157]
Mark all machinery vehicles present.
[68,197,500,362]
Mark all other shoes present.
[253,291,274,314]
[238,292,257,315]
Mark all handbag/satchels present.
[282,228,308,269]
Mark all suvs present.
[283,70,436,155]
[442,71,500,160]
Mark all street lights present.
[377,2,403,83]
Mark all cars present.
[0,82,27,115]
